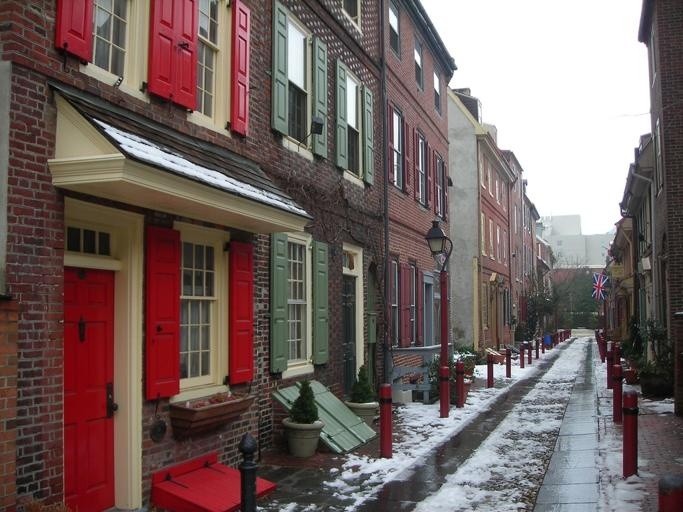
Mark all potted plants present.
[282,375,324,457]
[345,364,379,426]
[638,319,674,400]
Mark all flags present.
[590,271,608,301]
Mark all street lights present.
[425,220,453,365]
